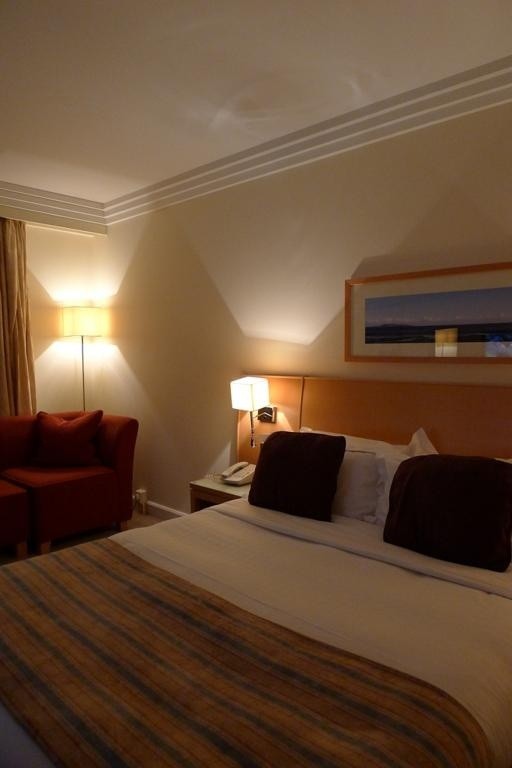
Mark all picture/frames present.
[343,262,511,364]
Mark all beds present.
[0,430,512,768]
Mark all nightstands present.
[183,475,250,517]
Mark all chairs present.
[1,411,140,563]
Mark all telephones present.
[222,461,256,485]
